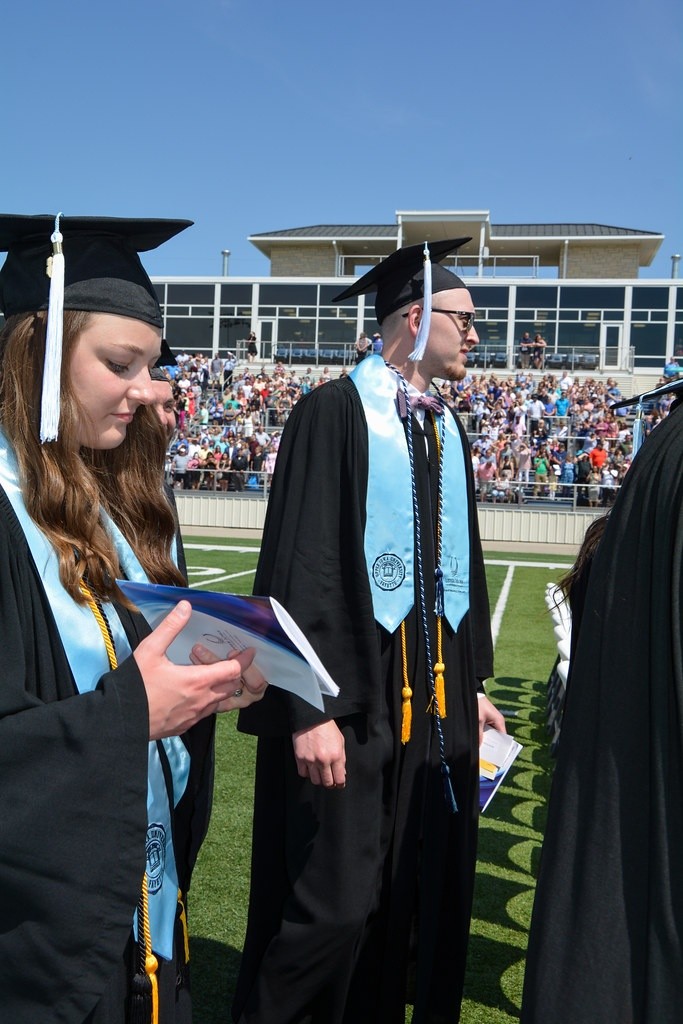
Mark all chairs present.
[463,352,596,369]
[272,348,358,365]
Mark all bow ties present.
[397,388,444,417]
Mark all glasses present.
[402,307,476,331]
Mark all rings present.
[234,689,242,696]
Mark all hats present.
[331,237,473,362]
[179,448,186,452]
[0,214,194,452]
[609,378,683,469]
[373,332,381,338]
[258,375,263,378]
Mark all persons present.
[247,331,257,362]
[372,332,384,355]
[0,213,266,1024]
[159,350,346,493]
[520,376,683,1024]
[231,237,507,1024]
[437,331,683,507]
[357,332,368,364]
